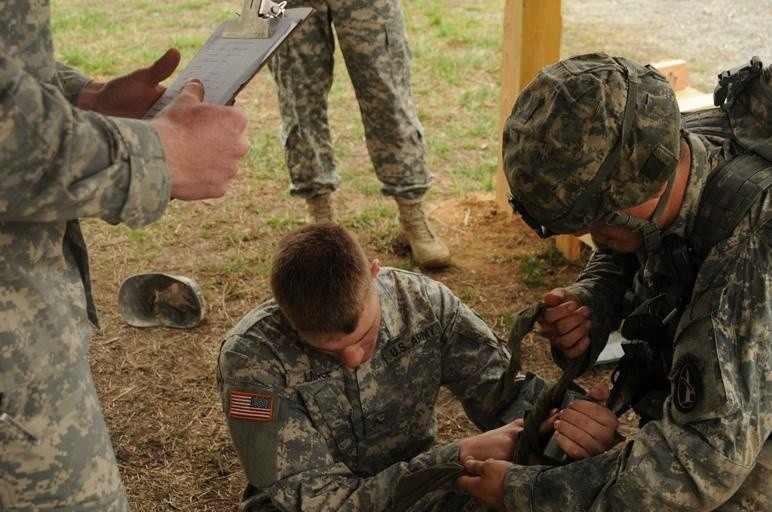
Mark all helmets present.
[502,53,679,239]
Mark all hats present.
[119,272,206,328]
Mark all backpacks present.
[684,57,772,258]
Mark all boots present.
[399,203,450,269]
[306,195,359,239]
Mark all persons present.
[217,220,619,512]
[0,1,249,512]
[263,0,451,269]
[456,53,772,512]
